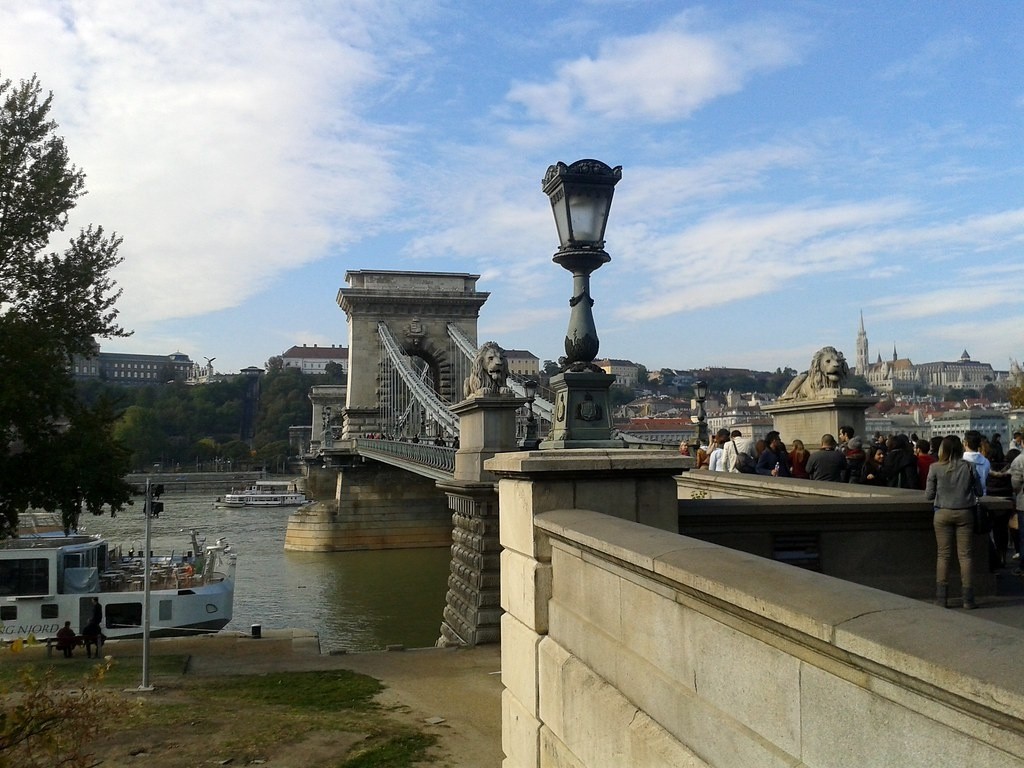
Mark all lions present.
[463,341,512,399]
[776,346,859,401]
[328,406,342,425]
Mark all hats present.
[849,436,862,451]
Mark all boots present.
[934,583,980,610]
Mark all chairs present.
[101,557,204,587]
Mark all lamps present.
[691,380,708,404]
[522,379,537,403]
[542,159,622,264]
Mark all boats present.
[2,492,241,646]
[211,478,311,509]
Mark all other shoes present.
[1013,552,1020,558]
[64,654,73,657]
[88,654,99,658]
[1010,566,1023,575]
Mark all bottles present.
[774,462,780,477]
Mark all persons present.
[364,430,461,450]
[55,620,76,658]
[83,617,102,660]
[1010,433,1024,576]
[90,597,108,646]
[694,426,1024,490]
[924,435,986,612]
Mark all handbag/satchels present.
[734,453,757,474]
[973,503,990,534]
